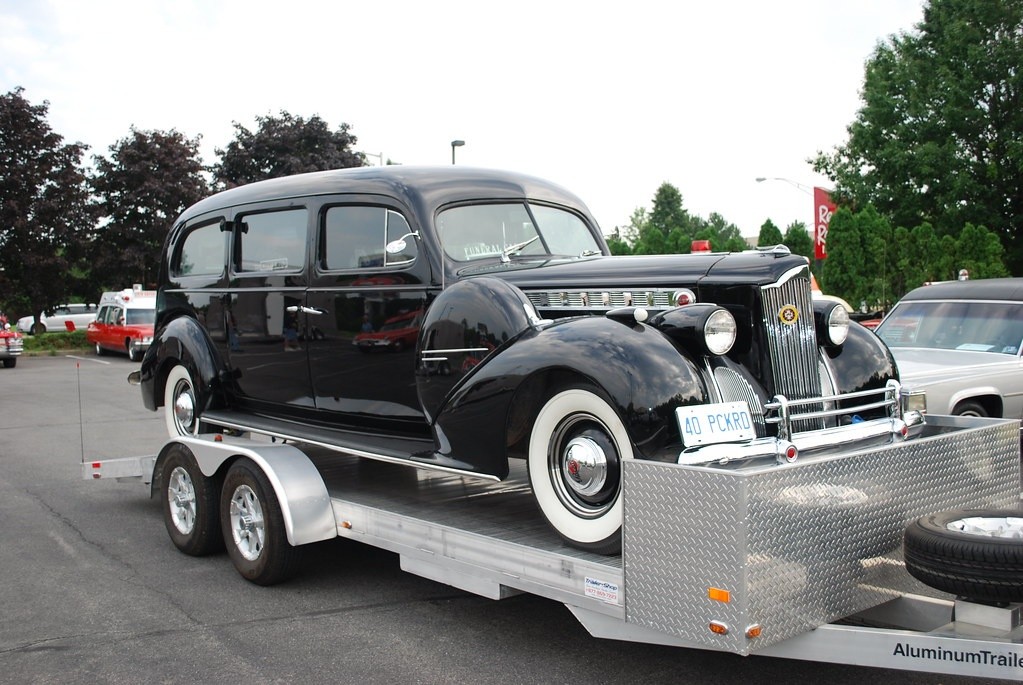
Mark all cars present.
[128,164,930,556]
[856,315,925,345]
[85,283,157,363]
[875,270,1023,443]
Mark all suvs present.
[15,302,97,334]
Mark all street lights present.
[452,140,465,163]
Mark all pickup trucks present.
[0,314,24,369]
